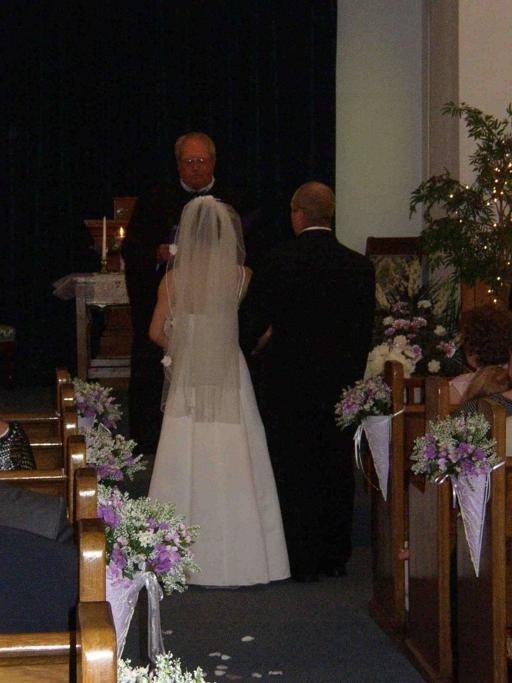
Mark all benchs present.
[362,361,510,681]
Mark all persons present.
[0,420,37,471]
[238,181,376,582]
[438,303,512,406]
[120,131,245,456]
[147,195,291,590]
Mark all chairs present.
[0,365,121,681]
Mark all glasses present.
[180,158,209,163]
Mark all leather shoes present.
[293,572,320,582]
[329,564,348,577]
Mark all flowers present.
[73,381,126,437]
[334,377,392,429]
[117,649,206,681]
[85,417,146,487]
[95,484,202,596]
